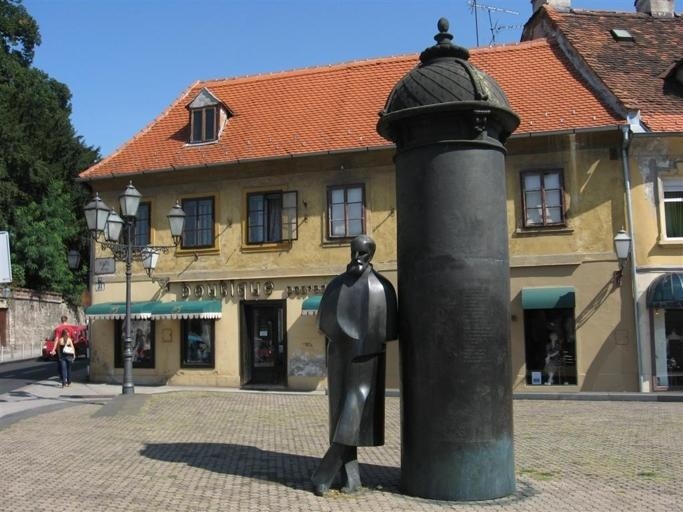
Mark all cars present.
[40,324,86,360]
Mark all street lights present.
[84,179,184,394]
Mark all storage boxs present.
[532,371,542,384]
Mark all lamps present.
[66,242,90,292]
[141,244,169,287]
[613,223,631,286]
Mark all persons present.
[58,329,75,388]
[133,328,145,361]
[50,315,76,383]
[311,235,399,497]
[544,333,561,386]
